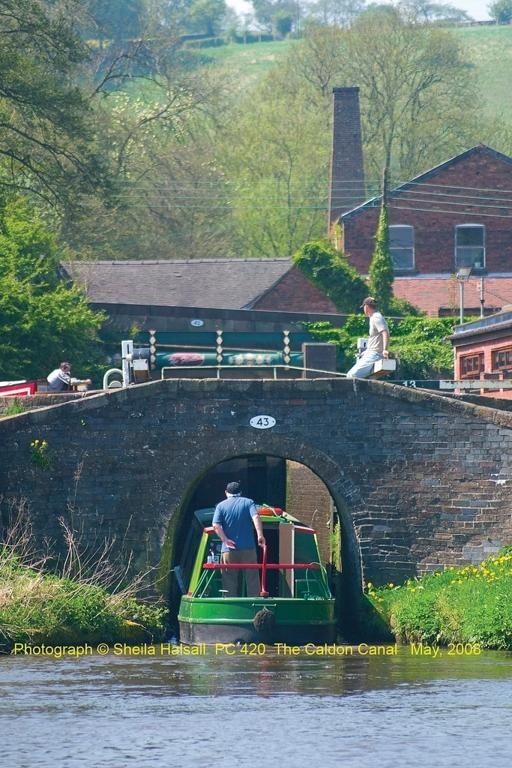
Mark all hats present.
[226,481,241,493]
[360,297,378,309]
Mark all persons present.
[346,295,391,380]
[46,359,93,392]
[211,480,269,599]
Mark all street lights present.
[452,265,472,327]
[469,268,490,318]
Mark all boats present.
[174,499,335,643]
